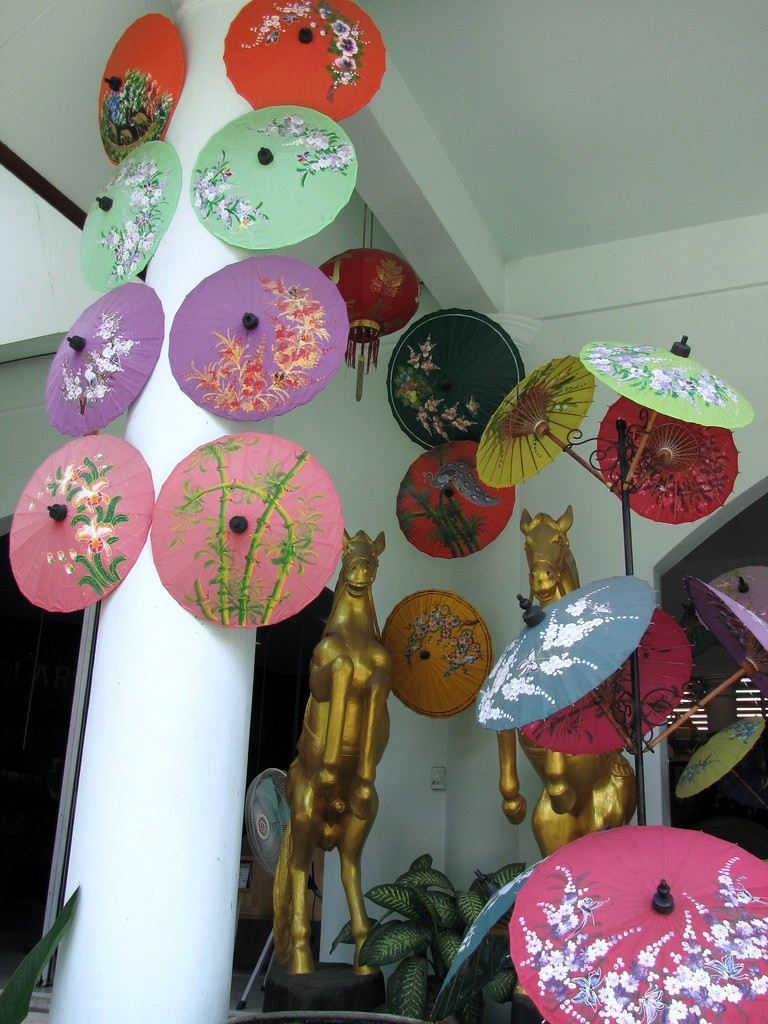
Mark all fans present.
[243,766,292,877]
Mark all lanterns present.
[318,248,421,402]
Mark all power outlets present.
[430,765,447,791]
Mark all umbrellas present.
[427,825,768,1024]
[9,0,768,805]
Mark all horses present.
[492,503,638,851]
[273,528,397,975]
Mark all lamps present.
[674,602,715,659]
